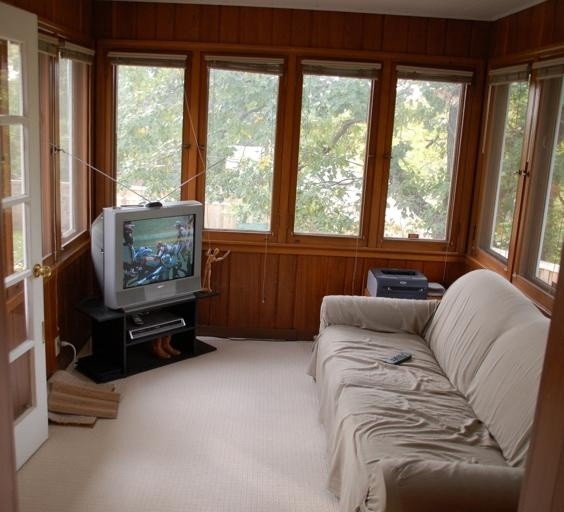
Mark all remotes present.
[385,353,412,365]
[133,316,146,326]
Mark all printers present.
[367,267,429,298]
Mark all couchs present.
[306,270,550,512]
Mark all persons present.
[153,334,181,359]
[121,215,194,286]
[202,247,231,293]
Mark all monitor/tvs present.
[90,200,204,313]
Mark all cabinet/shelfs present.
[75,293,218,384]
